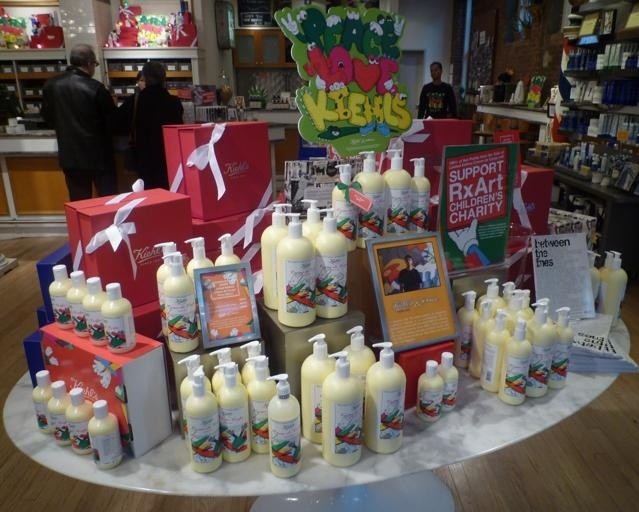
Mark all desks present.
[0,319,632,511]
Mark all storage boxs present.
[21,123,364,460]
[379,117,598,311]
[359,300,458,412]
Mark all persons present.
[116,61,184,191]
[40,43,123,203]
[136,71,146,91]
[417,62,458,119]
[494,73,512,102]
[398,255,422,293]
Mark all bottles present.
[439,352,458,414]
[416,359,444,422]
[544,123,552,144]
[48,265,137,355]
[587,114,638,142]
[548,85,559,117]
[560,143,633,186]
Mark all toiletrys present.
[417,352,458,424]
[331,162,360,252]
[561,42,639,173]
[80,276,106,347]
[301,326,406,466]
[261,199,348,330]
[410,156,431,235]
[352,150,385,250]
[456,278,574,407]
[47,264,73,330]
[153,234,242,354]
[177,340,301,479]
[101,281,136,355]
[65,270,89,338]
[586,249,629,333]
[381,149,410,239]
[151,199,459,482]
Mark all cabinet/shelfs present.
[3,45,199,227]
[552,168,638,288]
[546,1,639,196]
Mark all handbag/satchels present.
[114,136,135,151]
